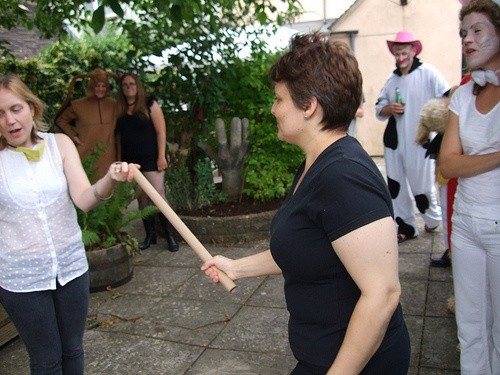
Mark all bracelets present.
[92,184,114,200]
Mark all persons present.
[430,67,472,263]
[439,0,500,375]
[55,67,124,184]
[376,31,446,242]
[201,26,411,375]
[115,72,179,253]
[347,95,366,138]
[0,73,141,375]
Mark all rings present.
[115,170,121,173]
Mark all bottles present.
[396,88,404,115]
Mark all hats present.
[387,31,422,56]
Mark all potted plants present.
[74,141,163,294]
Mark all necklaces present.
[127,101,136,105]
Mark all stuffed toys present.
[415,86,459,147]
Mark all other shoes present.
[397,233,407,242]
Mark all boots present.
[157,210,179,252]
[139,214,157,249]
